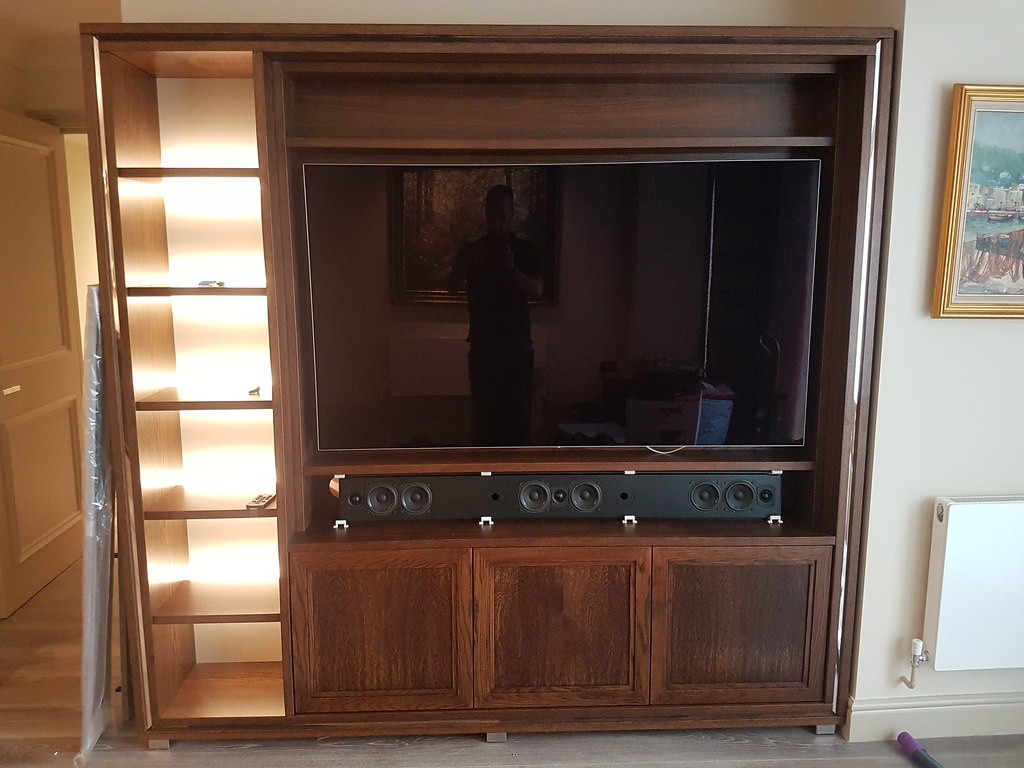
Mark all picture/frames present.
[931,84,1024,318]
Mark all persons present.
[438,184,543,447]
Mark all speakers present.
[335,474,783,522]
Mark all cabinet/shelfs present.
[287,514,836,737]
[79,23,295,749]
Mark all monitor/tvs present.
[287,151,824,451]
